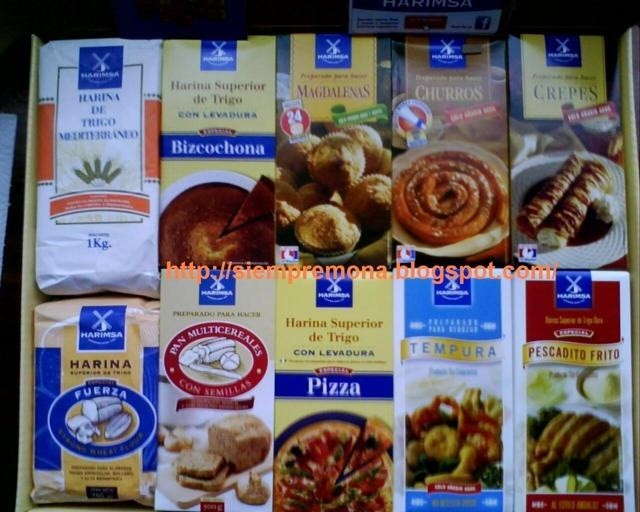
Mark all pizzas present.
[273,415,394,512]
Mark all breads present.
[174,414,271,492]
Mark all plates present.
[392,138,510,260]
[509,148,631,273]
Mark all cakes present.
[274,124,392,258]
